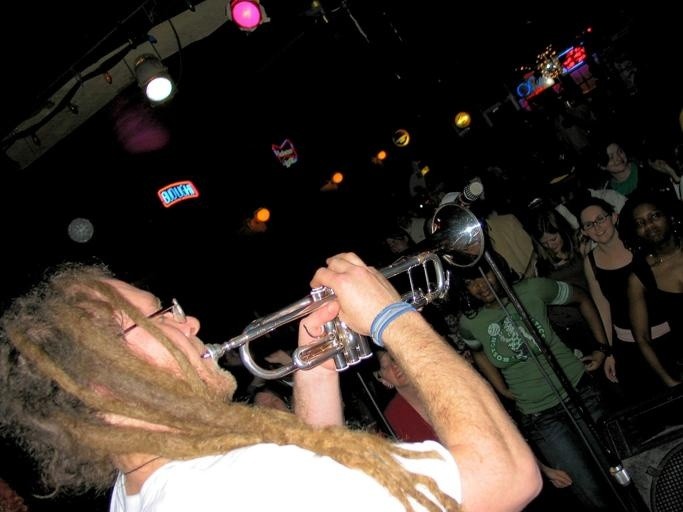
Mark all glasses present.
[108,296,188,347]
[580,213,612,234]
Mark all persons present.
[242,134,681,512]
[0,249,545,511]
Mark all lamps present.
[225,1,272,36]
[120,39,178,111]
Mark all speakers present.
[602,382,683,512]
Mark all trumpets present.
[206,202,485,380]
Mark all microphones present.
[454,181,484,209]
